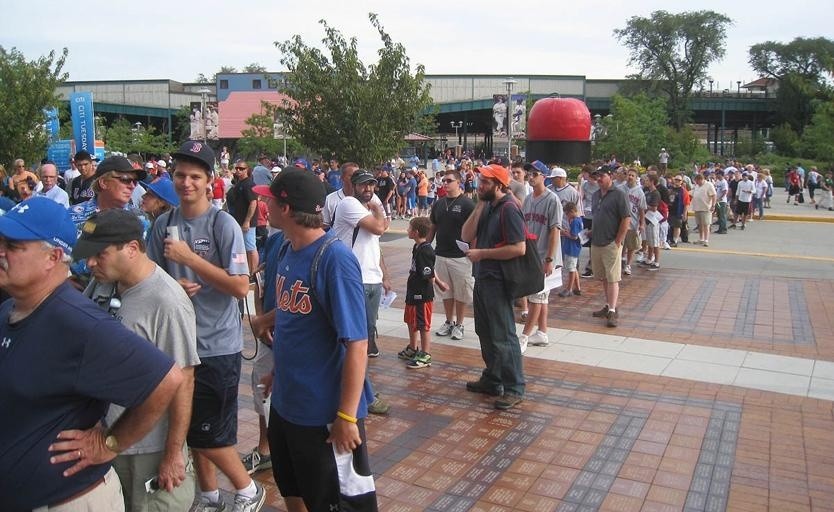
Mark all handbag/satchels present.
[495,201,544,302]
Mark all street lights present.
[450,120,463,157]
[594,114,601,120]
[197,82,212,145]
[606,115,614,120]
[502,75,518,164]
[135,121,141,135]
[735,80,742,96]
[708,79,715,96]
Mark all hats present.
[473,163,511,187]
[524,160,612,179]
[140,177,181,206]
[0,196,77,255]
[71,207,144,257]
[169,140,214,173]
[251,166,327,214]
[89,154,166,180]
[270,166,282,172]
[351,169,378,184]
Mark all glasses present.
[674,179,682,181]
[442,179,456,183]
[112,174,137,187]
[360,182,375,186]
[108,293,122,316]
[235,167,247,171]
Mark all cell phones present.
[151,479,159,490]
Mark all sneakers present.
[241,447,272,475]
[435,321,455,336]
[450,324,464,339]
[367,397,388,414]
[398,344,419,362]
[229,481,267,512]
[713,220,746,234]
[367,346,380,357]
[560,270,593,297]
[663,238,709,250]
[406,351,432,368]
[518,310,548,354]
[622,252,660,275]
[188,488,227,512]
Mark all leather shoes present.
[494,390,523,410]
[607,311,618,327]
[592,305,617,317]
[467,381,504,396]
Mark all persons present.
[0,139,396,512]
[191,104,202,138]
[783,162,834,211]
[396,143,774,409]
[493,96,508,135]
[510,99,524,132]
[206,105,218,137]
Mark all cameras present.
[26,175,36,190]
